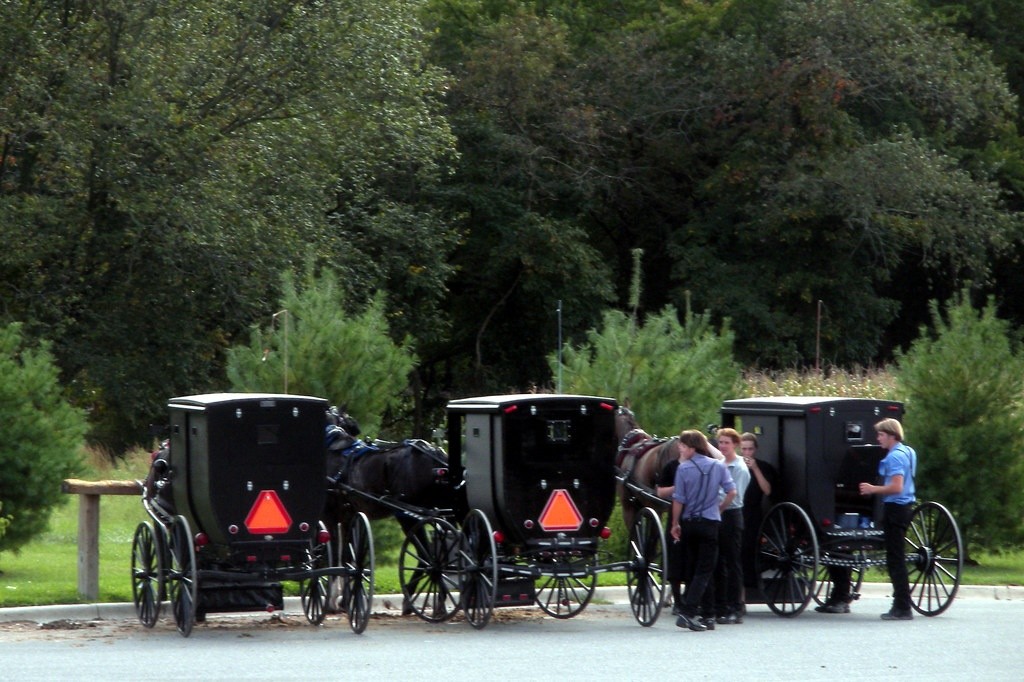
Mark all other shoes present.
[815,600,850,613]
[735,603,747,618]
[676,614,706,631]
[718,612,743,624]
[705,617,714,630]
[881,609,912,620]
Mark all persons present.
[815,549,853,612]
[657,427,779,631]
[859,419,916,620]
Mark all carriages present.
[318,392,622,631]
[612,396,965,629]
[129,391,376,638]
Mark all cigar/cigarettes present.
[674,540,677,544]
[745,458,749,461]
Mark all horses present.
[612,396,718,607]
[144,420,176,602]
[321,405,467,624]
